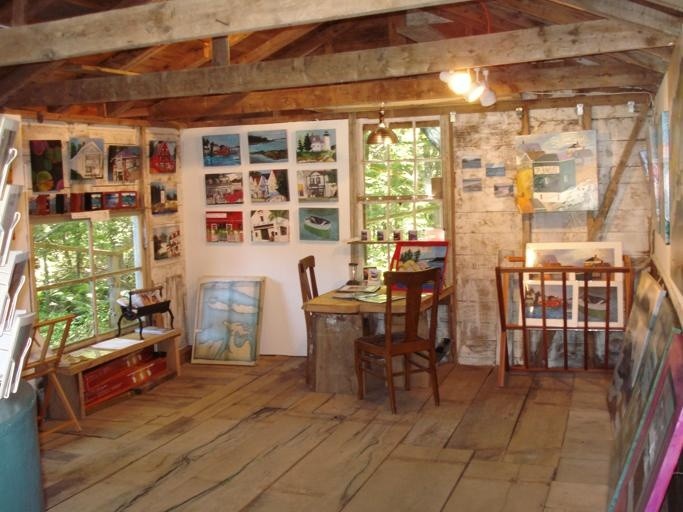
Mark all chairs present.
[354,268,442,415]
[299,256,318,392]
[22,314,81,438]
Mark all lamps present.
[439,68,497,107]
[366,110,398,144]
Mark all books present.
[332,292,379,299]
[0,147,35,400]
[335,284,380,293]
[354,294,406,305]
[135,326,175,336]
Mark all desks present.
[48,326,181,420]
[302,286,458,395]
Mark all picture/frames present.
[388,241,448,293]
[191,276,264,366]
[614,336,683,512]
